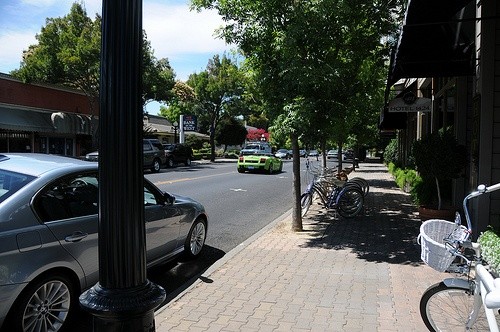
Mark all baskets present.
[420,220,467,273]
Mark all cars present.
[309,150,320,157]
[327,150,340,158]
[289,149,294,156]
[344,150,355,159]
[275,149,292,160]
[0,153,208,329]
[300,150,309,157]
[164,144,193,167]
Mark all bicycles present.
[301,159,370,219]
[414,183,500,331]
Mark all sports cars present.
[237,153,283,174]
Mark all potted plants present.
[412,130,468,223]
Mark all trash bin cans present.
[355,158,359,168]
[224,153,228,158]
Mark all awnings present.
[0,107,52,132]
[377,94,432,130]
[388,0,482,90]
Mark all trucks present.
[240,139,273,157]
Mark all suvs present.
[85,140,166,174]
